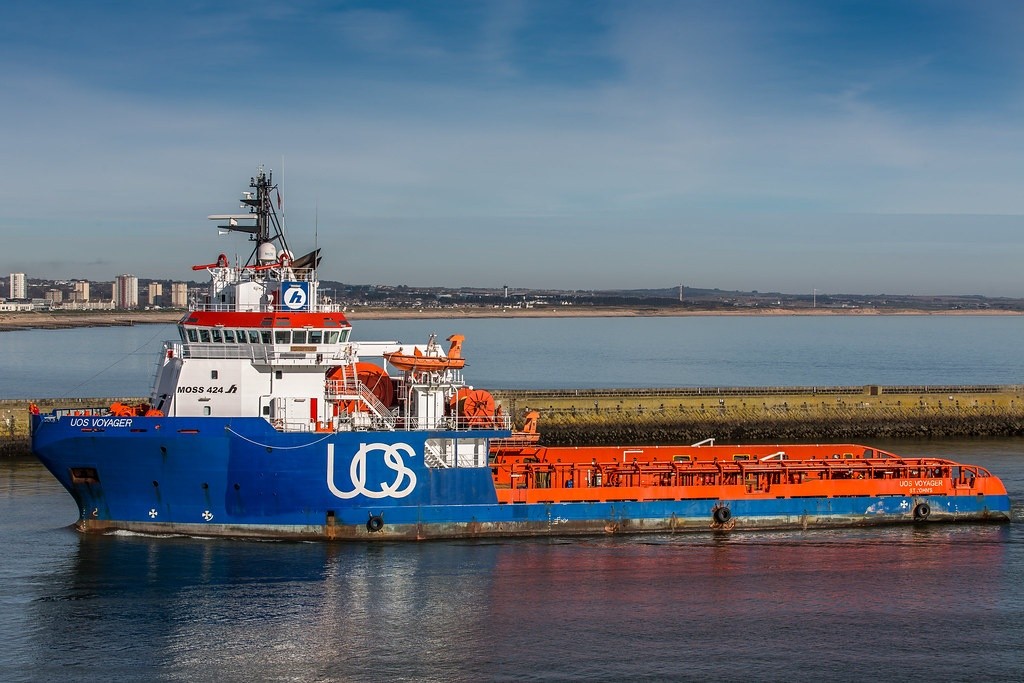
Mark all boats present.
[30,169,1010,542]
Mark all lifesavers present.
[716,507,731,523]
[916,504,930,518]
[369,516,383,530]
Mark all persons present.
[28,401,40,415]
